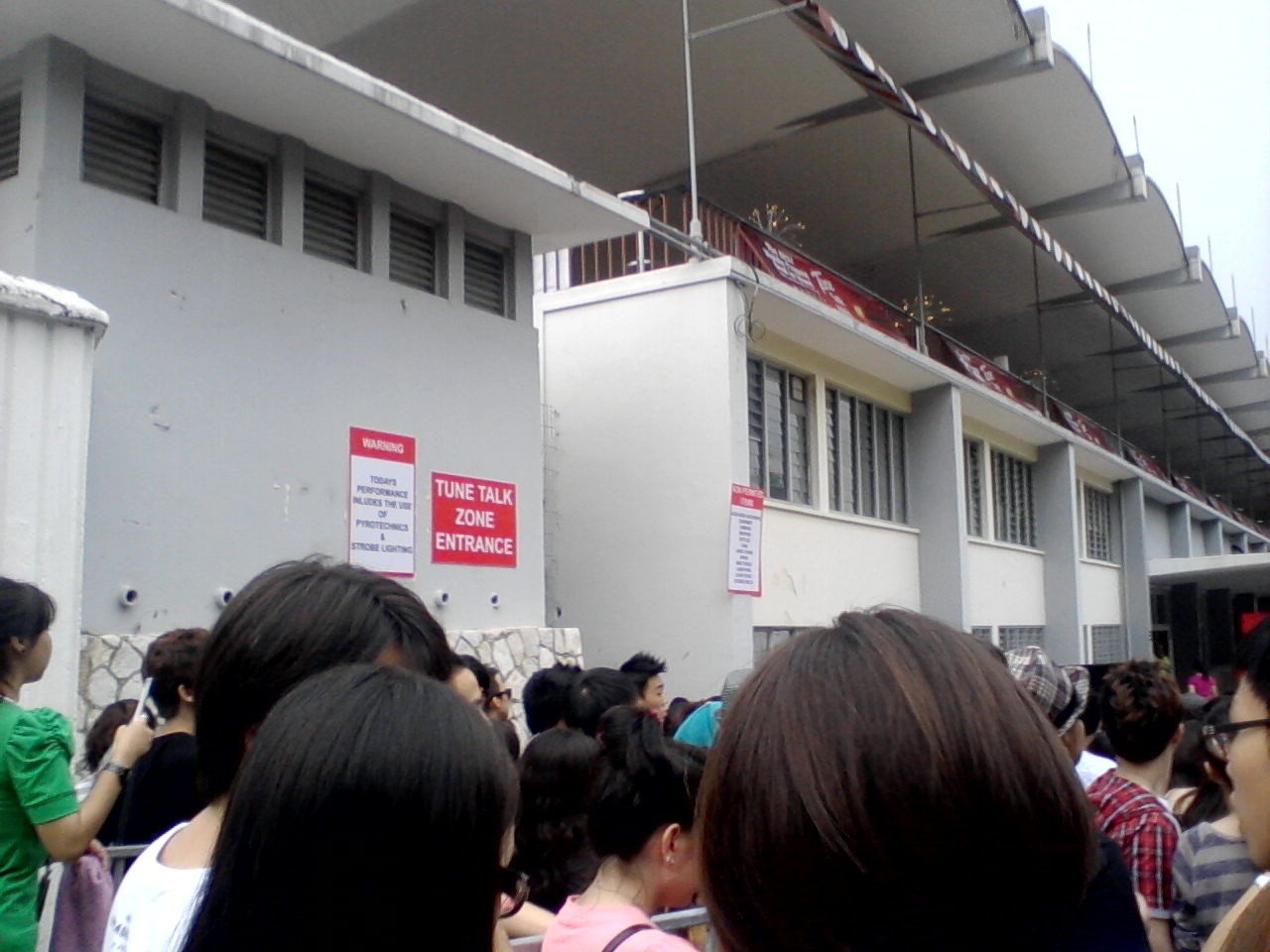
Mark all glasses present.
[1202,719,1266,758]
[499,867,528,921]
[487,688,511,704]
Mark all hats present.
[714,668,752,723]
[1002,643,1091,739]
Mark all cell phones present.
[128,677,154,725]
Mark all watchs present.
[103,761,132,785]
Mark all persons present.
[0,573,155,952]
[84,553,1270,952]
